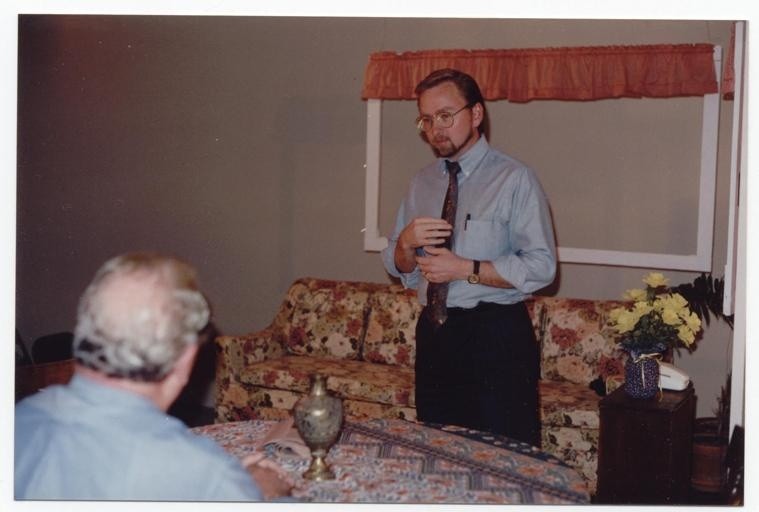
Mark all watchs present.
[468,259,480,284]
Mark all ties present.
[424,159,461,329]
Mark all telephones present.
[658,361,690,391]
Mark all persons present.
[15,249,292,501]
[381,68,558,448]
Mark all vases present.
[293,369,344,479]
[623,355,660,399]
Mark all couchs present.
[212,278,641,497]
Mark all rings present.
[425,272,430,280]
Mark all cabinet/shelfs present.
[598,387,694,504]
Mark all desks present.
[186,418,590,503]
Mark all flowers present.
[600,271,701,359]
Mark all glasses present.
[415,105,469,132]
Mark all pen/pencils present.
[464,214,471,231]
